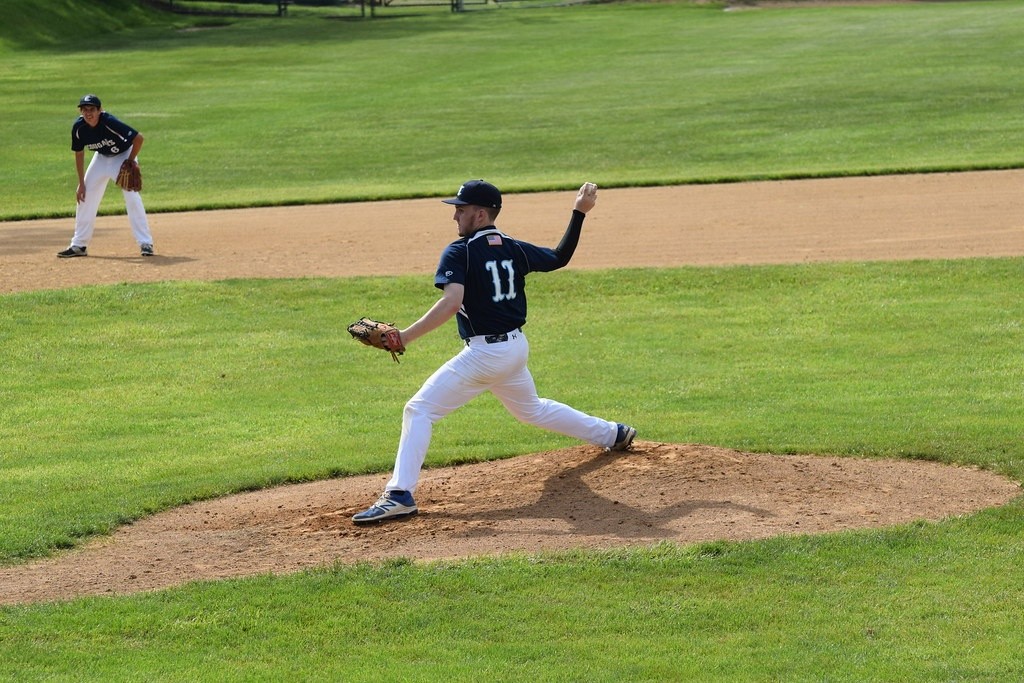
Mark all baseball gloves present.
[115,159,142,193]
[346,316,407,365]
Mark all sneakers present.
[351,490,418,522]
[141,244,153,256]
[610,423,636,451]
[57,246,87,258]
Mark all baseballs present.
[581,182,593,195]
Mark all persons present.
[347,179,637,523]
[57,94,155,257]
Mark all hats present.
[440,179,502,207]
[77,95,101,108]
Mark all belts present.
[466,327,521,343]
